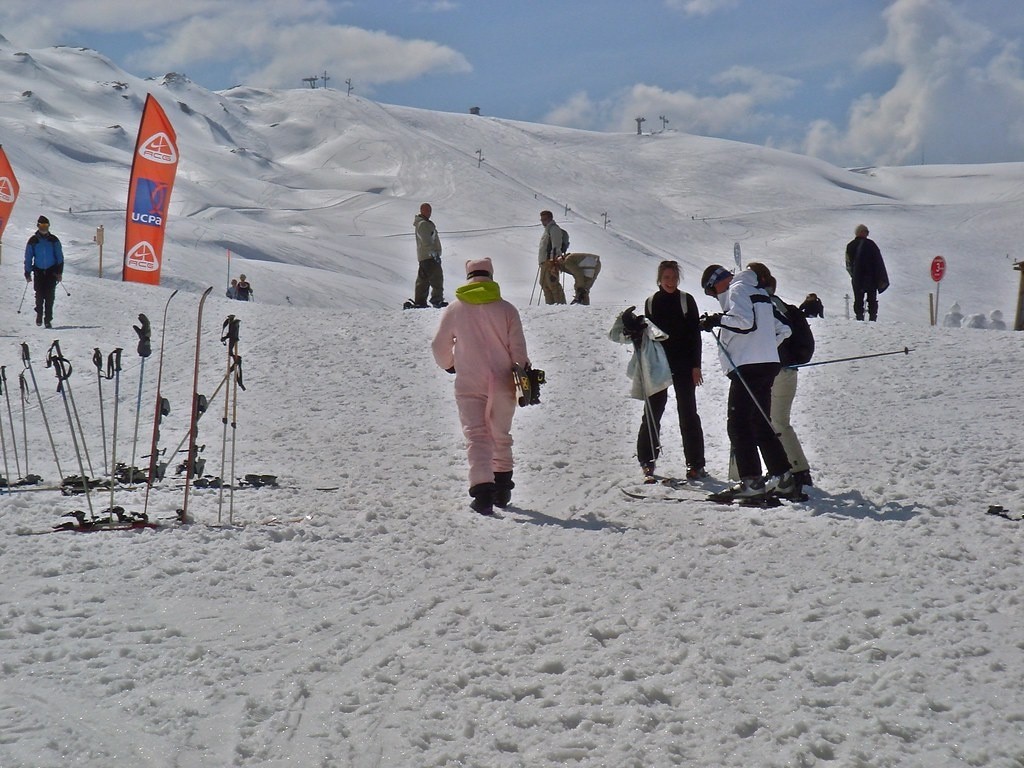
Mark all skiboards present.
[620,477,809,509]
[143,284,214,522]
[643,478,688,486]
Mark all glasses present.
[806,296,811,299]
[660,260,678,265]
[705,267,726,296]
[865,230,870,234]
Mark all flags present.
[0,143,19,242]
[122,93,180,286]
[25,215,64,327]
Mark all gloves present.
[699,314,713,332]
[132,314,151,358]
[622,306,648,343]
[445,365,456,374]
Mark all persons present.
[846,224,884,322]
[745,262,815,489]
[431,257,541,514]
[538,211,601,304]
[621,261,712,482]
[414,203,450,308]
[227,274,253,301]
[700,263,798,499]
[798,293,825,319]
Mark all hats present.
[38,216,49,223]
[702,264,728,288]
[465,258,496,278]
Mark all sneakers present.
[413,302,430,308]
[763,469,795,497]
[643,467,656,484]
[793,469,814,486]
[34,307,43,326]
[685,464,710,481]
[434,300,450,308]
[44,317,53,328]
[719,475,765,498]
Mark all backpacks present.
[548,224,570,253]
[769,295,814,367]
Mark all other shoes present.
[856,313,864,321]
[869,314,877,322]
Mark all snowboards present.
[512,361,531,407]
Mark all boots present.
[494,470,515,507]
[468,482,496,514]
